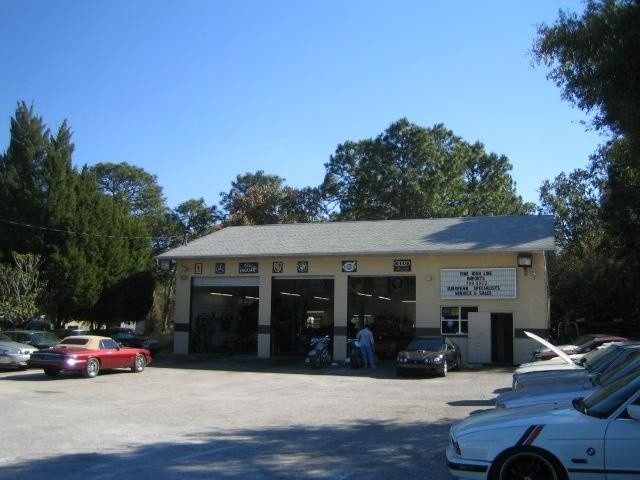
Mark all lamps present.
[159,259,177,274]
[517,254,533,268]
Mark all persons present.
[355,323,379,370]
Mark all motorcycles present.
[305,336,332,368]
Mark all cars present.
[393,334,465,378]
[445,330,639,480]
[0,327,161,379]
